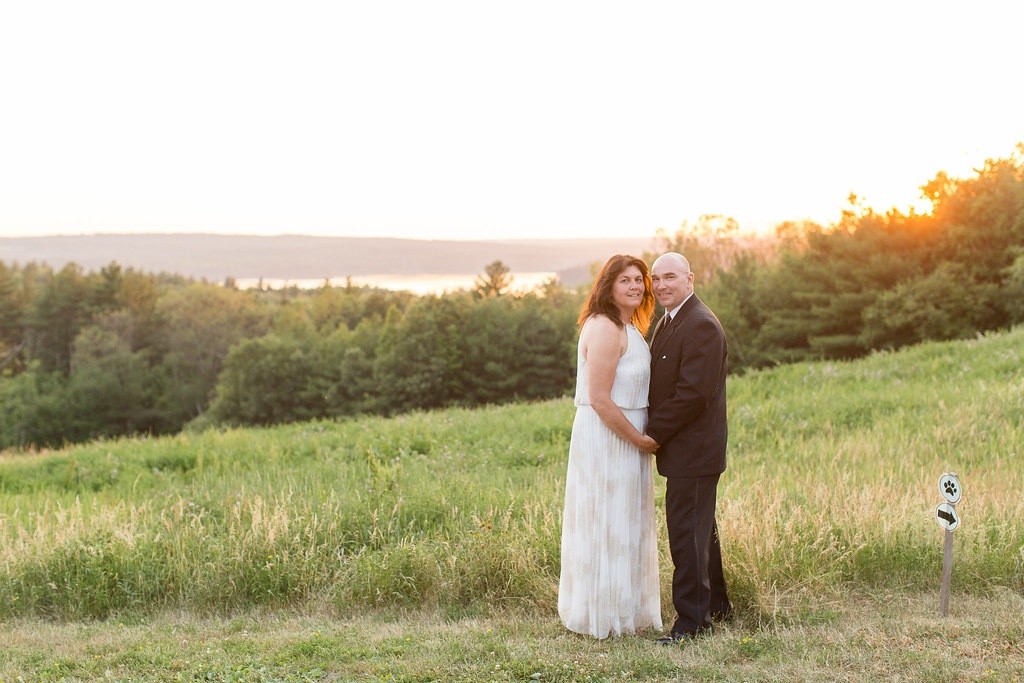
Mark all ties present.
[652,313,671,352]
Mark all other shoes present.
[655,636,696,648]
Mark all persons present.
[645,252,736,646]
[557,254,664,640]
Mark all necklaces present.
[620,319,637,330]
